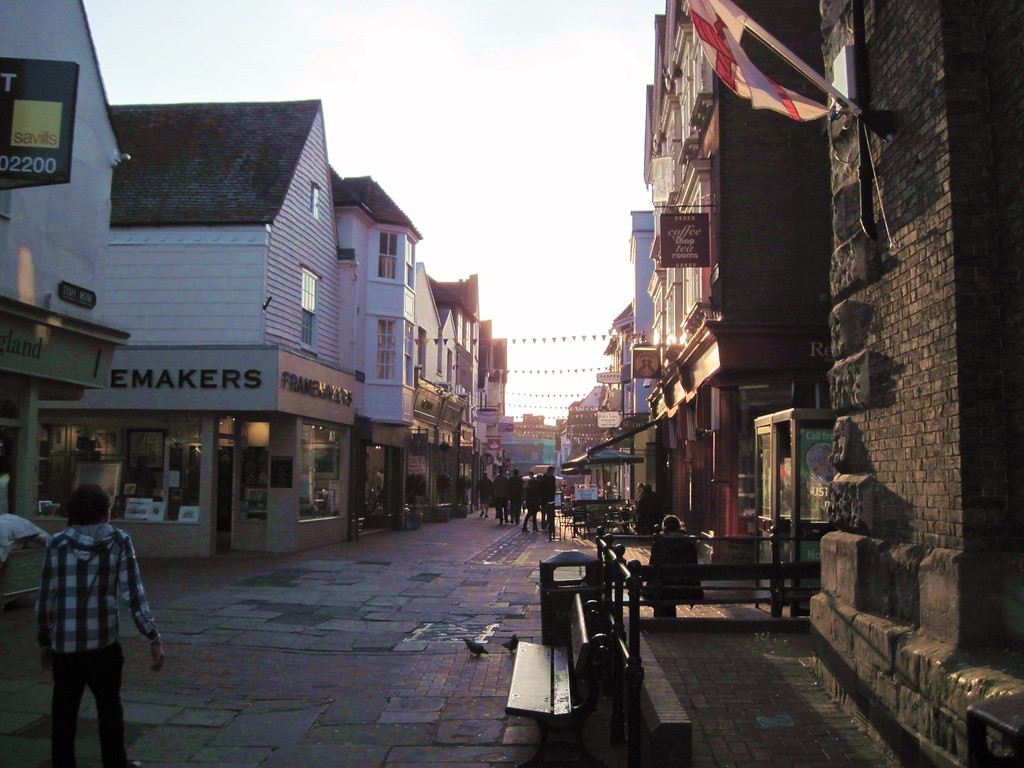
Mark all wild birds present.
[462,638,489,658]
[499,634,519,654]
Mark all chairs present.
[548,500,636,543]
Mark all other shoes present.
[127,759,142,768]
[532,528,541,532]
[480,512,484,516]
[42,759,53,768]
[485,515,488,518]
[522,528,530,533]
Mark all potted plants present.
[453,476,473,517]
[434,472,454,522]
[405,472,426,530]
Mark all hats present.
[664,516,681,528]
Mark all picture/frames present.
[298,442,339,481]
[314,489,335,516]
[127,428,167,471]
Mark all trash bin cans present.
[539,550,601,647]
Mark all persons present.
[477,467,557,534]
[644,515,705,601]
[631,482,664,530]
[36,484,164,768]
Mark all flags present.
[683,0,831,121]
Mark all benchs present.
[603,560,821,618]
[504,595,609,768]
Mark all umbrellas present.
[560,448,643,499]
